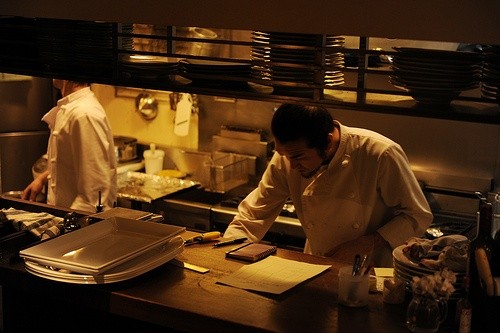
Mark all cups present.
[337,265,371,311]
[143,150,166,175]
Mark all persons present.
[21,78,118,213]
[222,101,433,262]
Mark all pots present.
[114,136,137,162]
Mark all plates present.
[122,54,252,86]
[247,30,346,95]
[90,206,154,220]
[389,42,500,107]
[390,244,465,300]
[17,217,186,288]
[0,15,132,75]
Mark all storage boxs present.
[202,153,250,194]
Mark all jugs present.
[405,283,447,332]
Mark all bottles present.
[470,204,500,276]
[456,277,472,333]
[60,211,82,235]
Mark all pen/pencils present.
[352,254,372,276]
[212,238,247,247]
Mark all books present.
[225,242,277,261]
